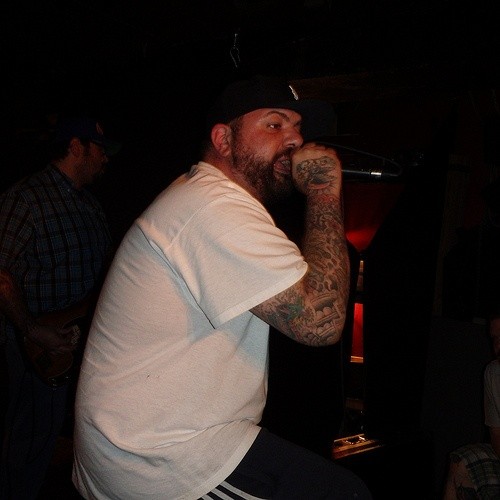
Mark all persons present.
[0,113,115,500]
[70,58,500,500]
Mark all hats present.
[57,117,119,144]
[215,77,328,124]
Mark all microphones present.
[337,166,394,183]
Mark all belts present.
[36,299,88,326]
